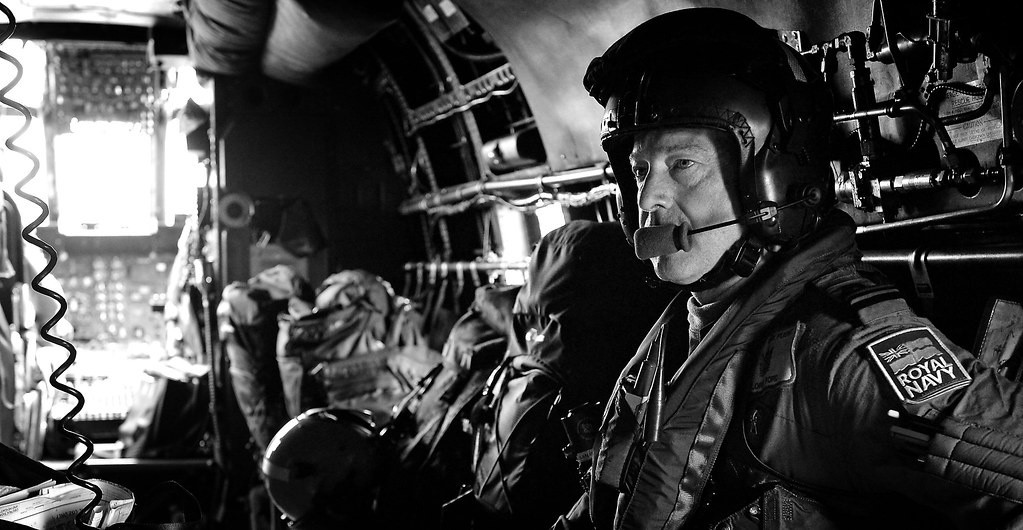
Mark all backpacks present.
[212,214,657,529]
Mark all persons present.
[551,7,1023,530]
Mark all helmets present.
[584,8,827,248]
[259,405,390,524]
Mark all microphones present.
[632,186,816,260]
